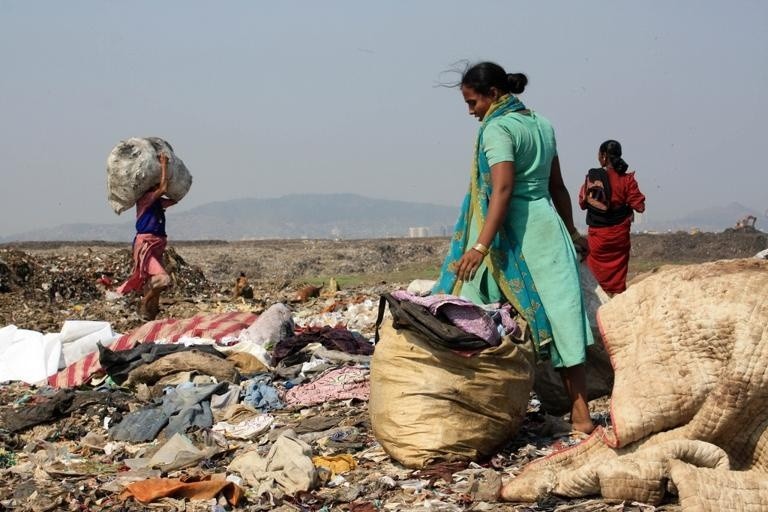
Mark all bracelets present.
[473,242,489,255]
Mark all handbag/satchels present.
[381,293,489,350]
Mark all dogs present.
[300,280,325,302]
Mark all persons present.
[120,151,171,319]
[580,140,646,297]
[431,62,595,435]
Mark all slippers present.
[137,313,153,322]
